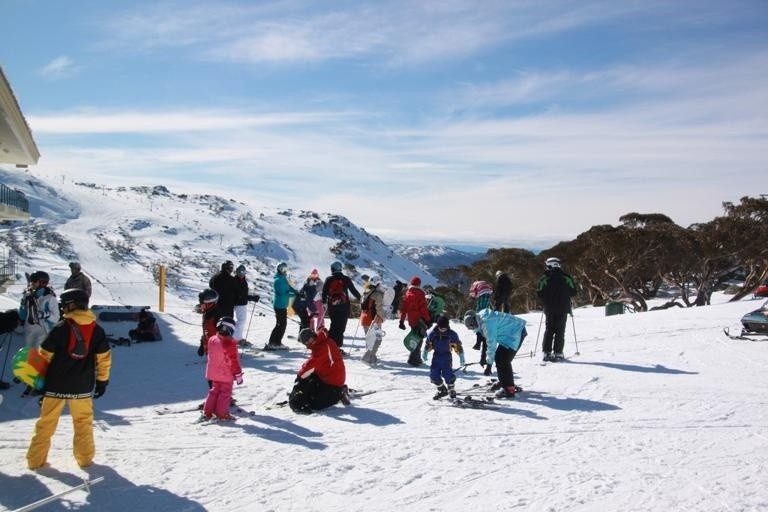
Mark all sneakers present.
[407,357,423,365]
[220,413,236,421]
[239,339,253,349]
[204,412,213,418]
[496,386,515,397]
[339,384,351,406]
[267,342,287,350]
[433,386,448,401]
[449,389,457,398]
[543,350,564,360]
[493,380,503,388]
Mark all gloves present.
[236,373,244,385]
[427,321,433,328]
[94,381,109,399]
[422,350,429,361]
[484,367,493,376]
[460,355,465,363]
[399,320,406,328]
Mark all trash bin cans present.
[604,300,624,316]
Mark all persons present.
[64,262,91,297]
[26,288,112,470]
[128,309,162,343]
[18,271,61,396]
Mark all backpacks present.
[288,377,317,414]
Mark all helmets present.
[495,270,504,280]
[30,272,50,282]
[69,262,81,270]
[216,316,236,333]
[464,314,473,330]
[437,316,450,328]
[221,260,247,276]
[297,328,312,344]
[60,288,89,305]
[464,310,476,317]
[331,262,343,275]
[276,262,288,274]
[368,274,381,288]
[544,258,562,272]
[198,288,220,303]
[410,276,422,286]
[310,269,319,279]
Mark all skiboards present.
[91,305,151,313]
[532,348,578,365]
[267,388,376,406]
[244,345,302,353]
[186,352,265,366]
[465,351,534,365]
[426,379,522,411]
[155,398,253,429]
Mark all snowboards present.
[403,297,446,353]
[365,288,397,350]
[11,345,49,391]
[99,312,147,321]
